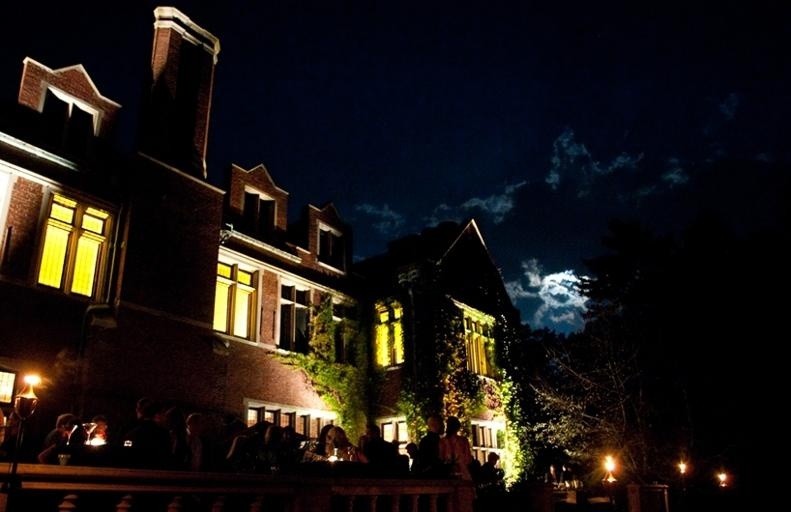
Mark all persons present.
[2,398,509,512]
